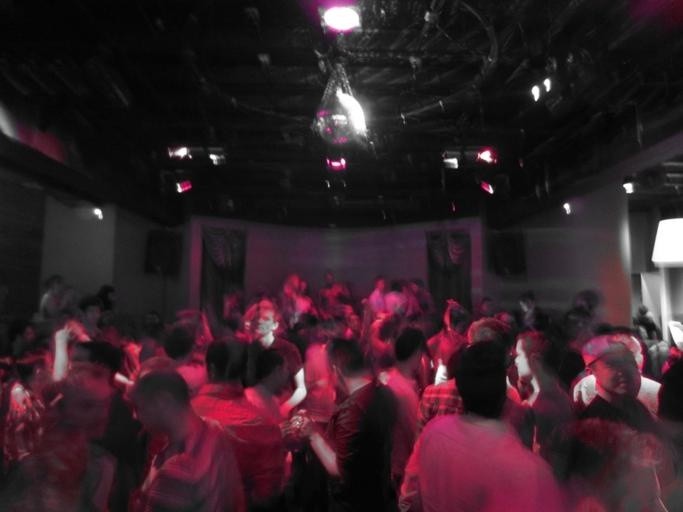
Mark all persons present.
[2,267,680,510]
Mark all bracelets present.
[18,452,30,461]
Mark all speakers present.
[144,229,180,276]
[493,230,527,276]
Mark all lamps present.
[312,62,367,144]
[650,218,683,265]
